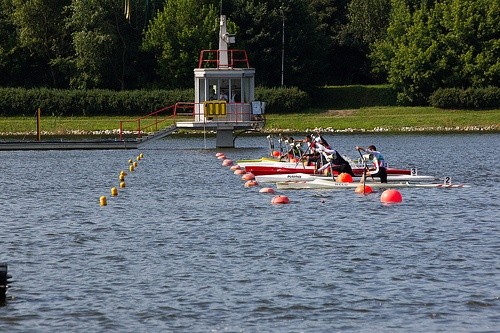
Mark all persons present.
[313,141,354,177]
[355,145,388,184]
[220,90,228,101]
[277,132,333,169]
[209,89,217,101]
[231,90,241,103]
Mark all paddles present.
[266,131,375,185]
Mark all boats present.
[277,179,457,192]
[254,174,433,182]
[237,157,416,175]
